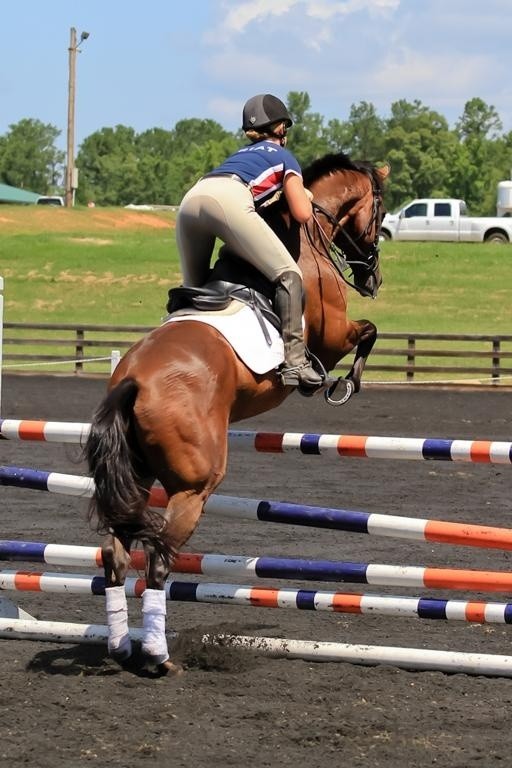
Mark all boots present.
[274,271,323,386]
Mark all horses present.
[75,150,387,675]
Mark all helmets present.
[242,94,292,129]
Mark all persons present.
[174,95,325,386]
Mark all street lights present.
[65,25,89,207]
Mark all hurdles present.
[1,421,512,675]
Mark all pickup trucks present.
[378,198,512,245]
